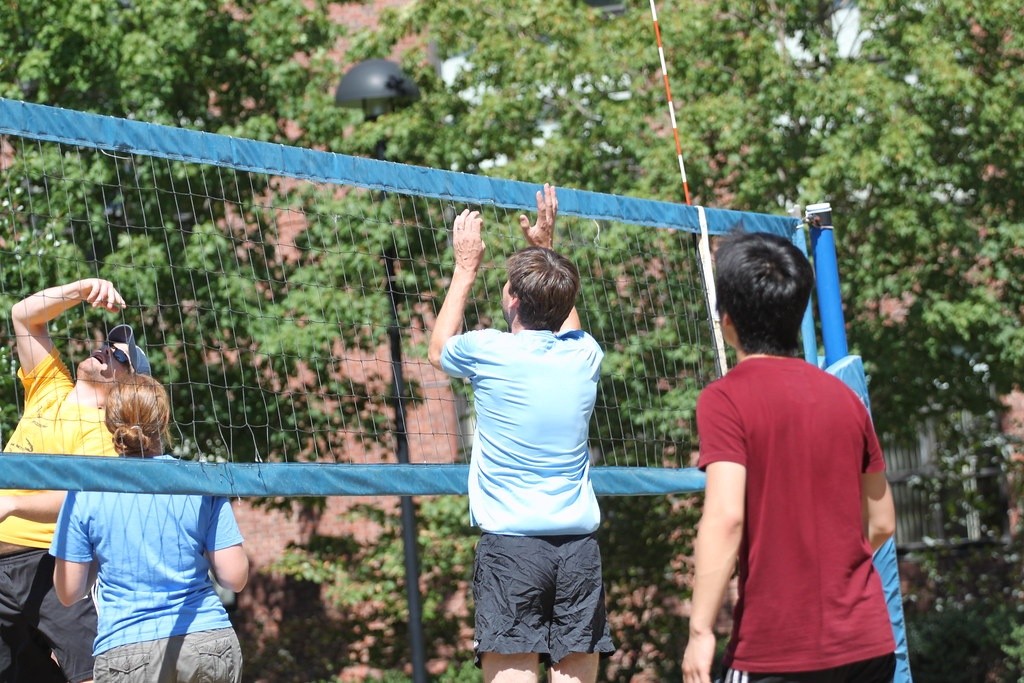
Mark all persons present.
[0,278,153,683]
[46,374,250,682]
[679,232,897,683]
[427,179,615,682]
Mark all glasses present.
[102,341,132,373]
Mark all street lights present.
[335,56,430,683]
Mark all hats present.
[105,324,152,376]
